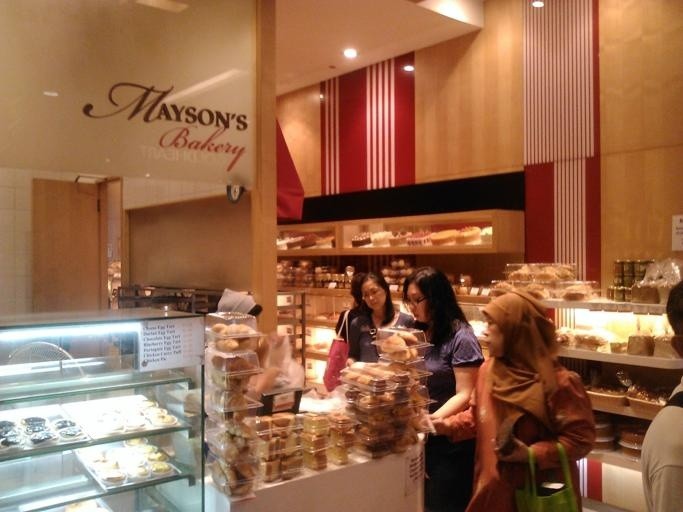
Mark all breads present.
[630,282,674,304]
[275,259,589,302]
[185,324,432,498]
[557,328,678,359]
[0,399,178,512]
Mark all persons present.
[402,265,484,512]
[638,279,683,512]
[345,272,416,366]
[420,288,598,512]
[335,271,365,342]
[217,288,263,323]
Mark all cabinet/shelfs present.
[276,209,525,394]
[277,292,306,388]
[542,298,683,512]
[0,307,206,512]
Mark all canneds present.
[607,259,655,302]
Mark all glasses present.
[405,296,426,302]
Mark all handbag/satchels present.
[515,442,581,512]
[323,310,350,392]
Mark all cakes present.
[277,226,493,248]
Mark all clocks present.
[226,185,243,203]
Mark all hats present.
[216,288,256,314]
